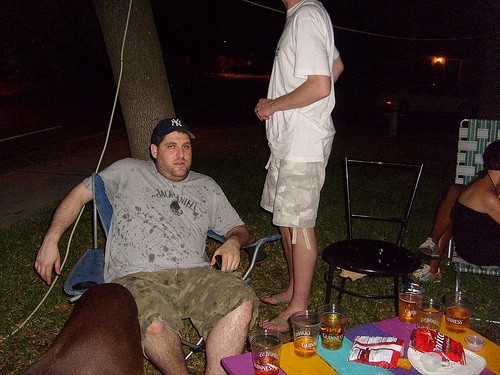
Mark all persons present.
[407,183,463,283]
[34,118,255,375]
[254,0,345,333]
[452,140,500,266]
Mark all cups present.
[398,282,427,323]
[290,310,324,358]
[248,329,286,375]
[445,291,475,333]
[318,303,349,351]
[413,299,447,332]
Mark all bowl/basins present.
[465,335,486,351]
[419,352,442,372]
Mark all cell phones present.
[215,255,222,267]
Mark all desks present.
[221,314,500,375]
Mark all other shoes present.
[417,236,441,258]
[408,263,443,284]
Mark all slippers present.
[260,292,290,305]
[259,314,292,333]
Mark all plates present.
[407,347,487,375]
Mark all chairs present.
[64,172,283,362]
[446,119,500,323]
[323,153,423,316]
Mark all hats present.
[483,139,500,170]
[153,116,195,141]
